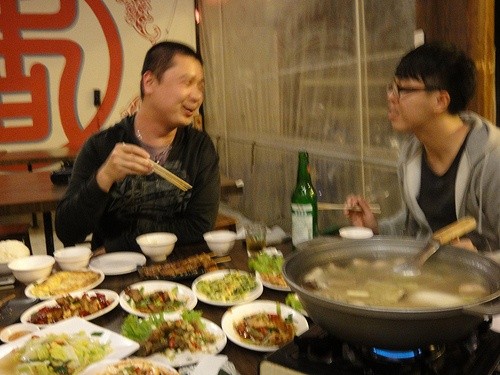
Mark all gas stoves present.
[259,324,500,375]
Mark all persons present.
[342,40,500,254]
[54,41,221,263]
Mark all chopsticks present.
[149,159,192,192]
[317,203,381,213]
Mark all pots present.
[281,235,500,350]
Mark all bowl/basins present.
[203,230,237,256]
[0,249,30,275]
[136,232,178,261]
[0,323,40,344]
[340,225,373,240]
[7,254,55,284]
[53,246,92,271]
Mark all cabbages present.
[12,330,111,375]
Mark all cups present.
[243,224,268,257]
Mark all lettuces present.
[286,293,303,322]
[120,308,207,343]
[248,253,284,275]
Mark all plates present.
[221,300,309,352]
[24,267,106,300]
[119,280,197,317]
[0,316,141,375]
[191,269,264,306]
[89,251,147,275]
[125,318,227,368]
[20,288,120,328]
[85,360,180,375]
[255,269,293,291]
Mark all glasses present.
[384,80,441,105]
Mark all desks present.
[0,146,245,256]
[0,241,295,375]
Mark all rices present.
[0,240,30,265]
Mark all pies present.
[30,270,102,296]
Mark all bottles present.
[290,151,318,251]
